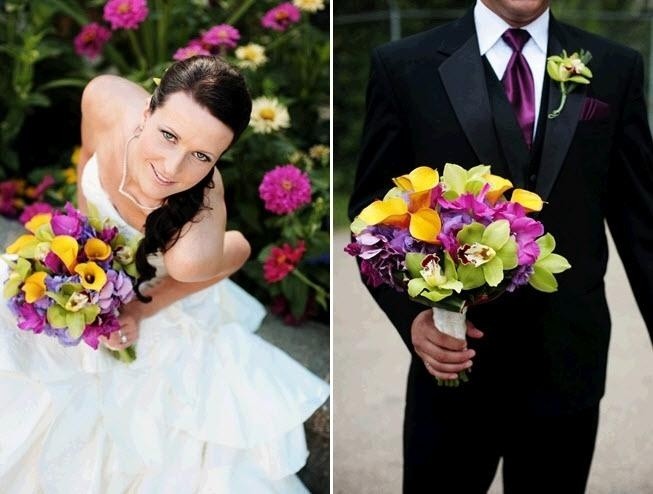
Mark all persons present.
[347,0,653,494]
[0,53,330,494]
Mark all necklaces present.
[118,134,165,210]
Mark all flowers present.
[345,163,570,385]
[0,202,144,361]
[547,49,594,120]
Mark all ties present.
[499,28,537,151]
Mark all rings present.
[122,336,127,343]
[118,330,122,338]
